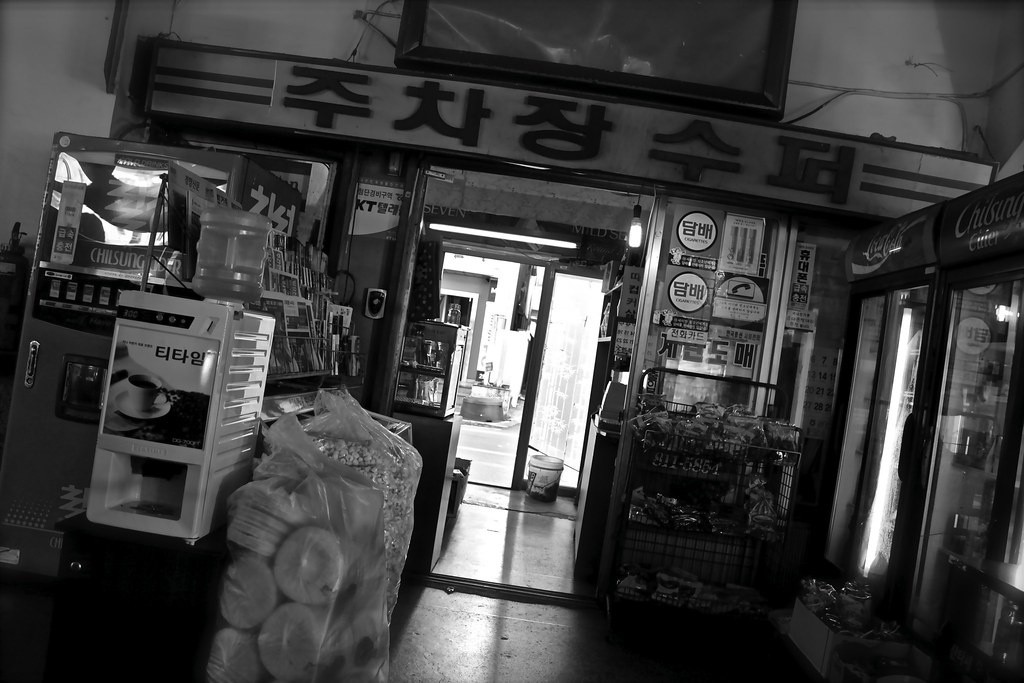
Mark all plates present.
[113,390,171,419]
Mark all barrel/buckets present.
[526,454,564,503]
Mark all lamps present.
[427,221,581,250]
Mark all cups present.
[127,374,170,411]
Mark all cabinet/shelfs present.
[603,394,804,642]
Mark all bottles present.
[191,207,273,305]
[447,304,461,326]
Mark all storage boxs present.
[787,596,910,679]
[447,458,472,518]
